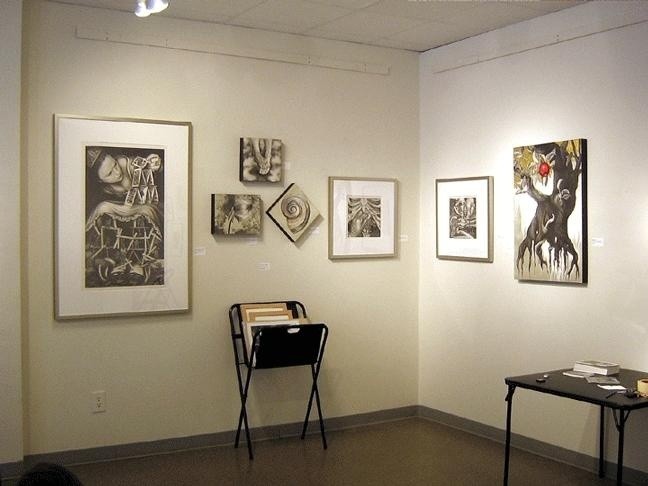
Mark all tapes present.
[637,378,648,394]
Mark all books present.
[563,370,594,378]
[585,376,621,385]
[573,358,622,377]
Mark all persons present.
[251,138,273,176]
[85,147,164,286]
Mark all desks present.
[502,368,648,486]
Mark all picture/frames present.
[434,175,495,264]
[51,112,194,322]
[326,175,400,260]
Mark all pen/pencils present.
[606,391,617,399]
